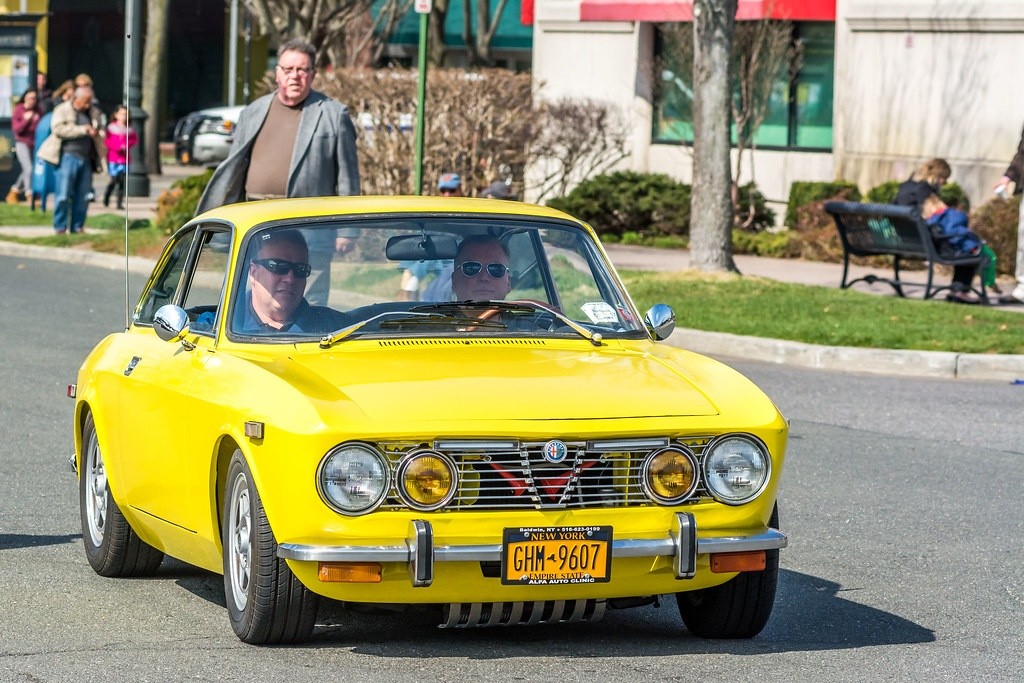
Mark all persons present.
[192,39,359,308]
[894,128,1024,304]
[190,227,358,340]
[5,72,139,234]
[397,173,541,332]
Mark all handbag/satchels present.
[927,222,983,259]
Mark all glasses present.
[252,258,311,277]
[455,261,510,279]
[275,64,314,74]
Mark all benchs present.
[824,200,990,306]
[348,301,439,323]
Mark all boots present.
[5,185,20,205]
[24,191,39,206]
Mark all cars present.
[172,84,415,168]
[65,195,792,642]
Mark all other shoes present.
[952,293,979,304]
[998,295,1020,303]
[945,293,954,300]
[988,284,1002,293]
